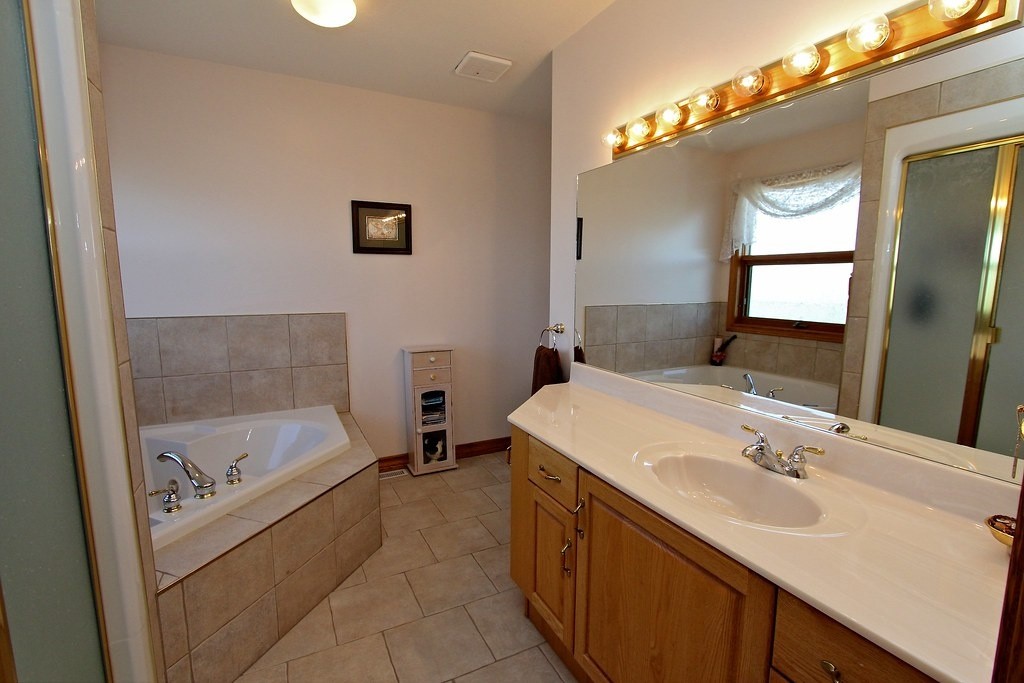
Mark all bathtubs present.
[137,405,383,681]
[622,364,839,415]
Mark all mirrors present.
[572,23,1023,487]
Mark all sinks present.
[774,413,977,491]
[630,441,867,539]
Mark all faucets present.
[156,452,216,499]
[751,443,786,474]
[828,422,850,435]
[742,374,757,395]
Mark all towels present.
[574,346,586,365]
[531,346,568,399]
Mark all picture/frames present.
[350,199,413,255]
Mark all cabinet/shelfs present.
[510,424,576,679]
[768,585,938,683]
[571,464,777,683]
[400,343,459,477]
